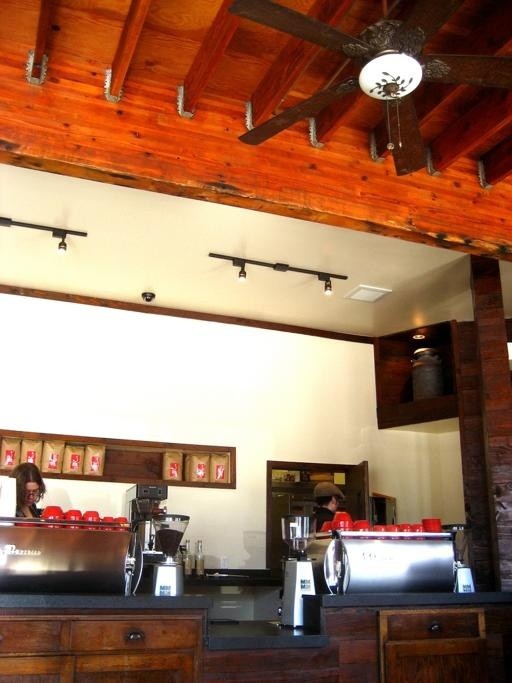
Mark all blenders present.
[278,513,317,629]
[150,513,191,596]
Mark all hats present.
[314,482,344,500]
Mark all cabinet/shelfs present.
[0,609,207,683]
[379,606,488,683]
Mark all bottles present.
[195,539,205,576]
[184,539,192,574]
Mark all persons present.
[308,482,346,540]
[9,462,44,518]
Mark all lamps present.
[59,237,66,254]
[239,267,246,282]
[325,281,332,295]
[358,49,423,101]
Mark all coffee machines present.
[123,483,169,562]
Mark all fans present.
[228,0,512,175]
